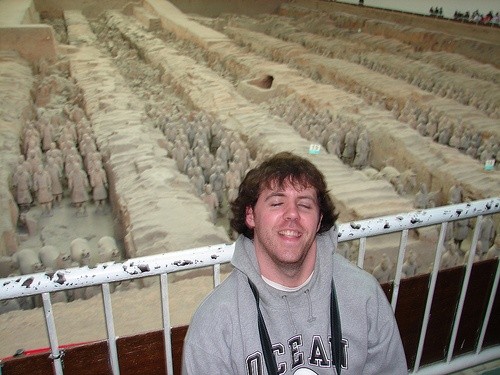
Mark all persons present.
[429,6,500,26]
[179,151,409,375]
[0,57,500,311]
